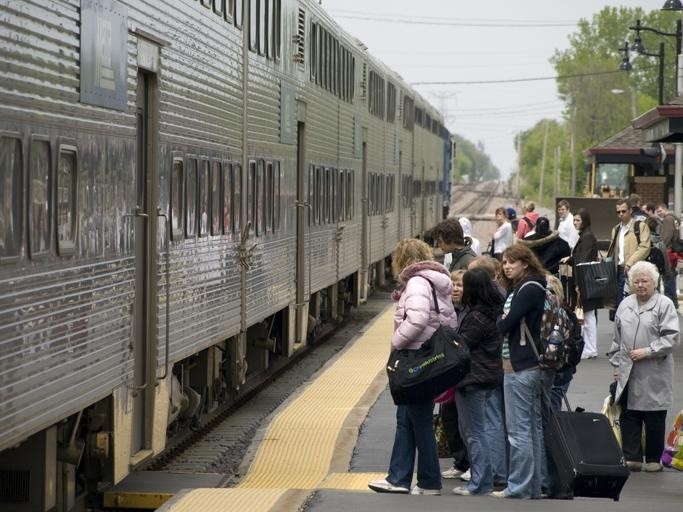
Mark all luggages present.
[545,386,629,501]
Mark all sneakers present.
[441,466,506,497]
[627,461,662,472]
[368,480,441,495]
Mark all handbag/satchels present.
[601,396,647,455]
[387,324,470,405]
[576,257,618,312]
[645,248,665,274]
[433,414,453,458]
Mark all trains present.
[0,0,455,512]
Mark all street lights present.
[610,86,636,117]
[626,16,681,98]
[617,39,667,107]
[660,0,683,13]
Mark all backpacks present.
[517,281,584,369]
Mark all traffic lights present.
[638,141,667,170]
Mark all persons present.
[366,235,457,496]
[422,193,683,499]
